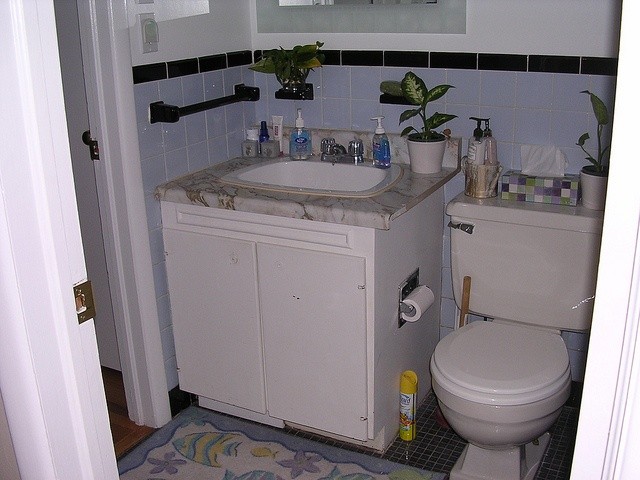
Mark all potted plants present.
[378,71,461,175]
[574,89,610,210]
[246,41,326,93]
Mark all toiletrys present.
[259,120,270,155]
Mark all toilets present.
[430,191,605,480]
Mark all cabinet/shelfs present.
[159,188,447,452]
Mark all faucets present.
[320,137,364,163]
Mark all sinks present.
[219,156,404,199]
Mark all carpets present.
[117,405,448,480]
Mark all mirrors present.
[256,0,466,36]
